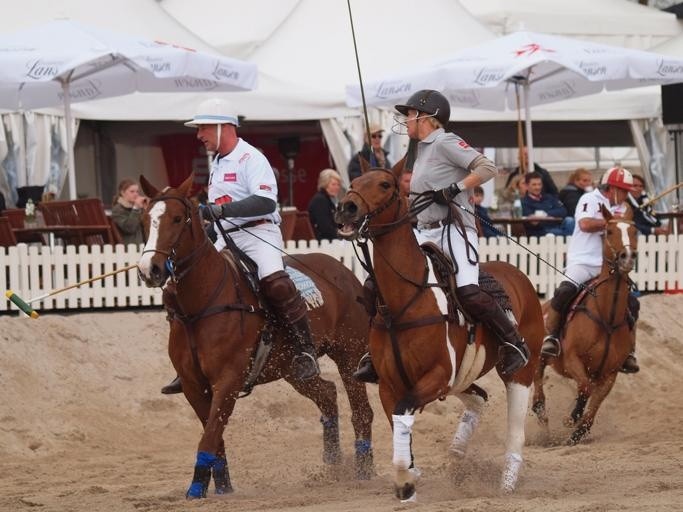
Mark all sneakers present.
[354,361,380,384]
[160,374,184,394]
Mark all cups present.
[534,210,543,217]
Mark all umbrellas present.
[344,32,682,174]
[0,17,258,200]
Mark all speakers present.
[278,136,299,157]
[661,82,683,125]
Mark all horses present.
[530,203,639,446]
[132,169,376,501]
[332,151,546,506]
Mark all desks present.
[11,224,112,245]
[653,212,683,234]
[491,217,563,244]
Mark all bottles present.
[672,190,679,214]
[512,190,523,219]
[24,198,34,224]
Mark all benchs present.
[1,207,39,228]
[37,198,126,252]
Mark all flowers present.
[25,199,35,223]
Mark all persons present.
[110,177,151,250]
[347,123,391,182]
[540,167,641,373]
[398,173,412,197]
[308,168,344,245]
[352,89,533,383]
[468,144,671,242]
[160,98,322,394]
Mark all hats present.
[394,88,451,127]
[598,166,635,192]
[183,97,241,130]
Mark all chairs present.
[280,212,297,248]
[0,217,17,246]
[292,211,316,247]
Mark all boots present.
[481,303,531,376]
[538,303,563,357]
[284,314,320,380]
[623,320,641,370]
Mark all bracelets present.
[456,181,466,193]
